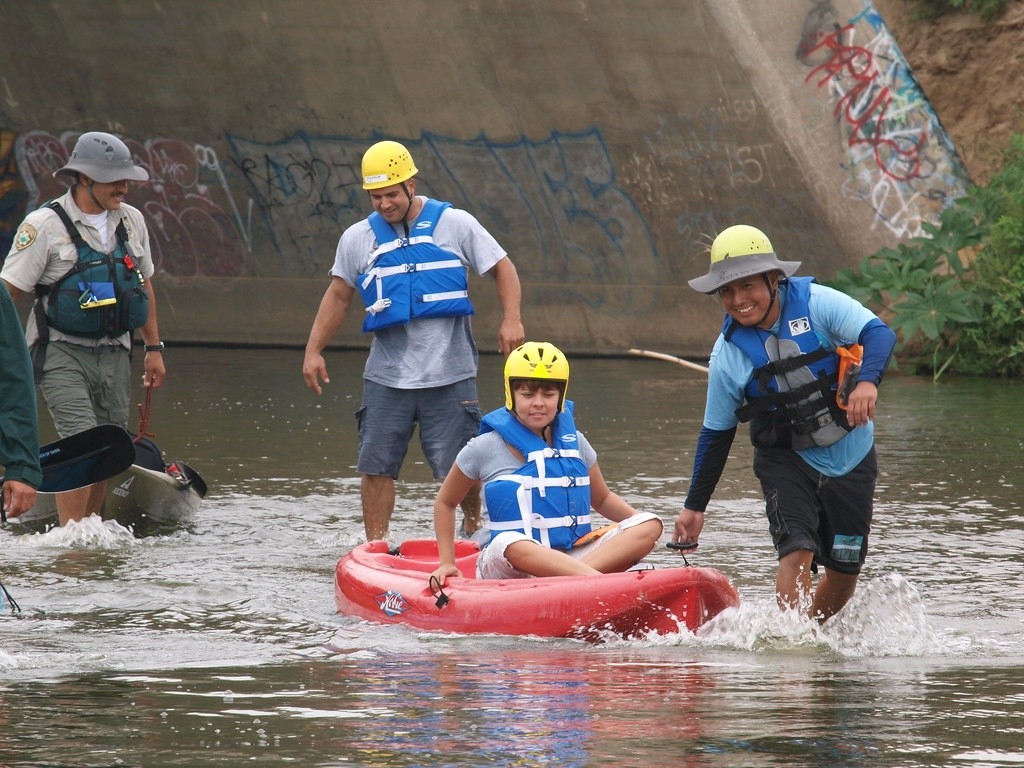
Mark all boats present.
[332,538,739,639]
[1,464,198,530]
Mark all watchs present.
[143,341,165,353]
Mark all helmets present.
[362,141,419,189]
[687,224,802,294]
[502,342,570,413]
[52,131,149,184]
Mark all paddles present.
[33,423,137,493]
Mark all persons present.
[429,339,664,594]
[0,131,167,529]
[0,278,43,519]
[670,223,898,625]
[302,139,526,542]
